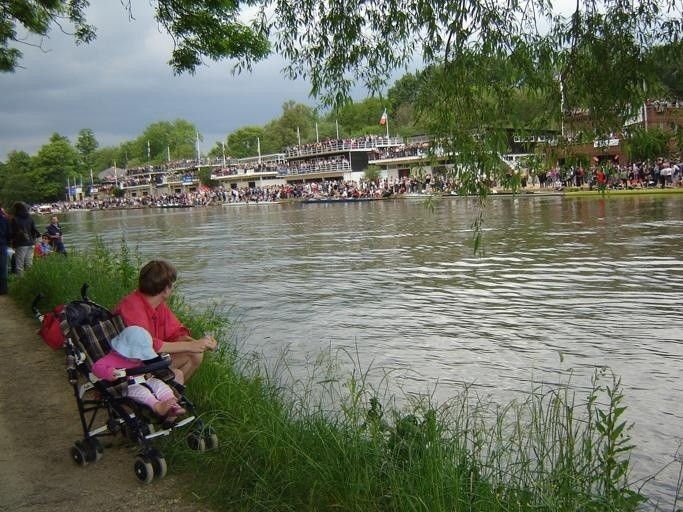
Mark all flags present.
[379,107,387,124]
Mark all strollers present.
[33,282,219,483]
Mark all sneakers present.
[168,403,185,415]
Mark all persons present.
[0,202,68,296]
[111,260,218,385]
[91,325,185,431]
[562,97,682,118]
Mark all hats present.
[111,325,159,361]
